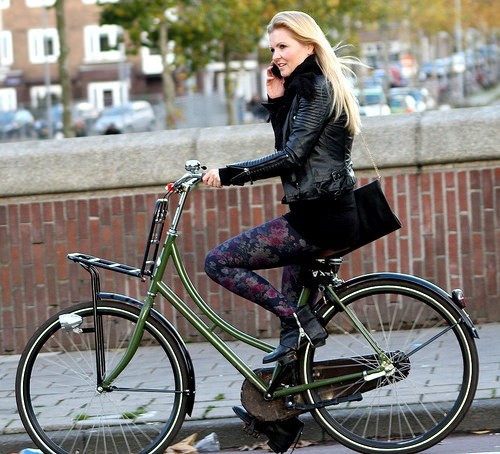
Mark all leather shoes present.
[234,408,304,453]
[262,303,327,365]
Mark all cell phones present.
[271,63,283,80]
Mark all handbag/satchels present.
[288,180,404,257]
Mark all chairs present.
[65,196,170,388]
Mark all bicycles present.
[12,159,482,454]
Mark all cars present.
[351,43,499,115]
[0,101,160,140]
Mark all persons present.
[200,10,373,454]
